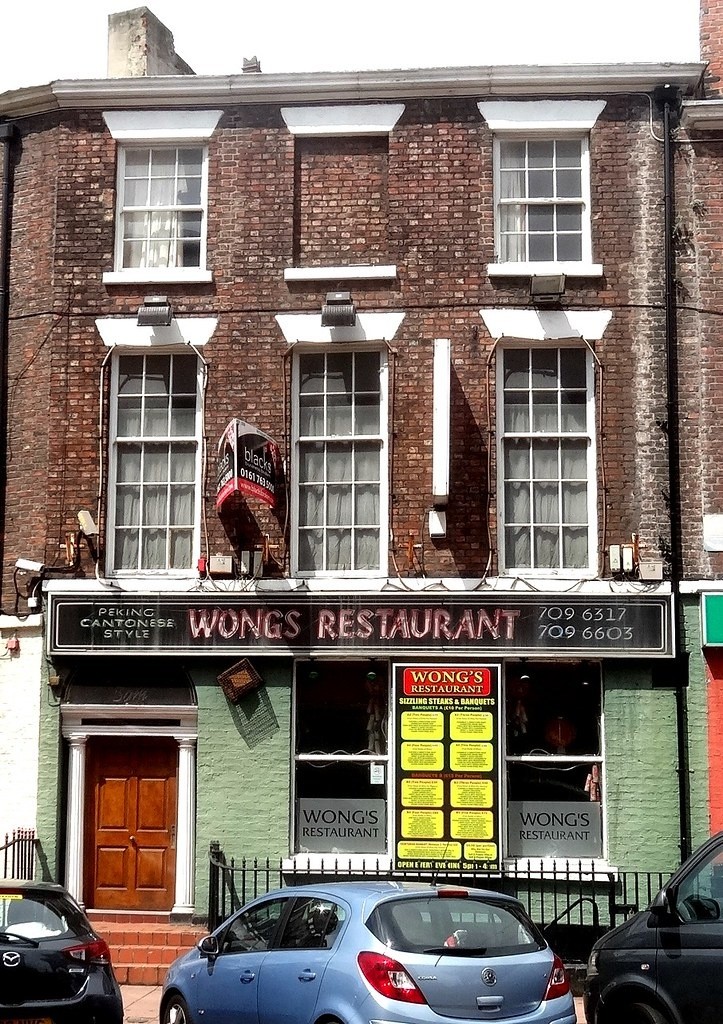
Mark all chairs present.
[0,898,37,934]
[297,906,436,949]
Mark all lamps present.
[529,273,567,303]
[136,295,176,327]
[321,291,357,327]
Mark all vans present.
[583,831,723,1024]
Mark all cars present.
[0,877,126,1023]
[159,880,576,1024]
